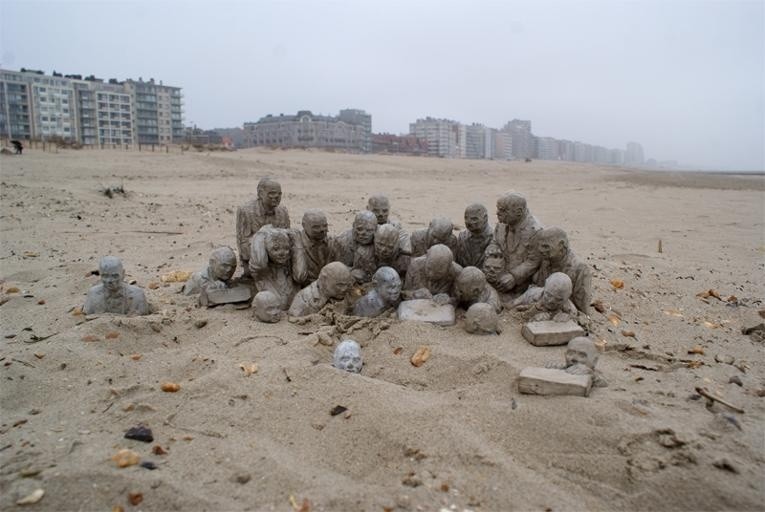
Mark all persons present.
[333,339,364,374]
[505,270,578,326]
[183,247,238,296]
[252,290,281,324]
[562,337,610,386]
[454,265,501,316]
[349,265,404,319]
[236,175,412,308]
[402,242,463,309]
[82,255,153,315]
[409,203,495,270]
[491,190,593,313]
[464,302,498,335]
[288,262,352,321]
